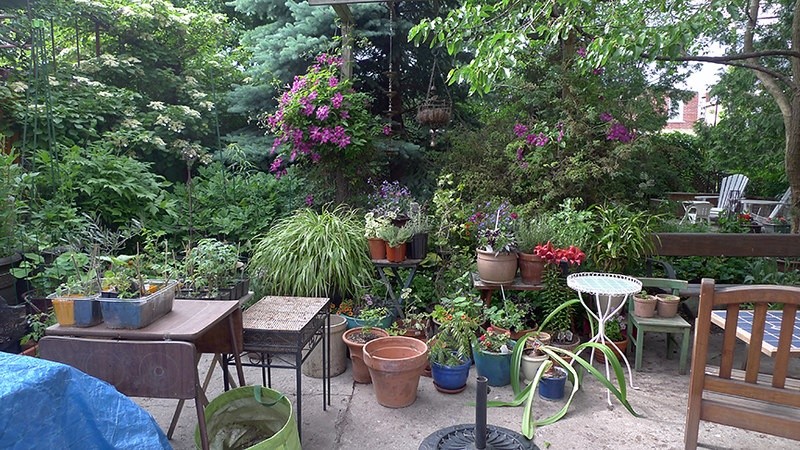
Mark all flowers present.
[765,214,789,225]
[477,222,626,349]
[738,210,759,225]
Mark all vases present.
[475,246,519,286]
[474,340,518,387]
[588,333,630,364]
[538,366,567,401]
[301,309,426,408]
[738,222,786,234]
[547,337,581,354]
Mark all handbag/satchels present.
[195,385,302,450]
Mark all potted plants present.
[364,212,429,262]
[431,218,548,392]
[0,133,250,358]
[635,291,680,318]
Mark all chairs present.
[679,174,791,228]
[684,279,800,450]
[627,276,691,375]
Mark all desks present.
[45,299,244,450]
[222,296,331,445]
[711,310,800,358]
[567,272,643,406]
